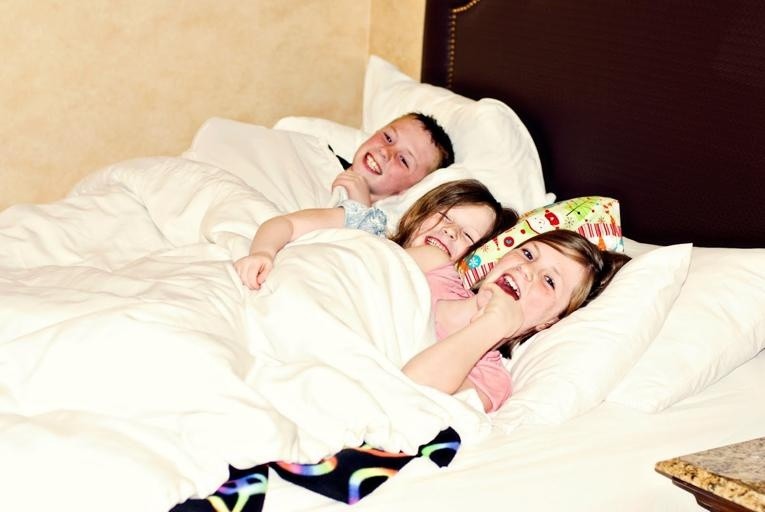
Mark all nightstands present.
[655,437,765,512]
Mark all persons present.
[325,111,453,211]
[233,177,519,290]
[400,228,635,413]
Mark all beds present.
[0,0,764,512]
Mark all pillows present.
[272,55,764,433]
